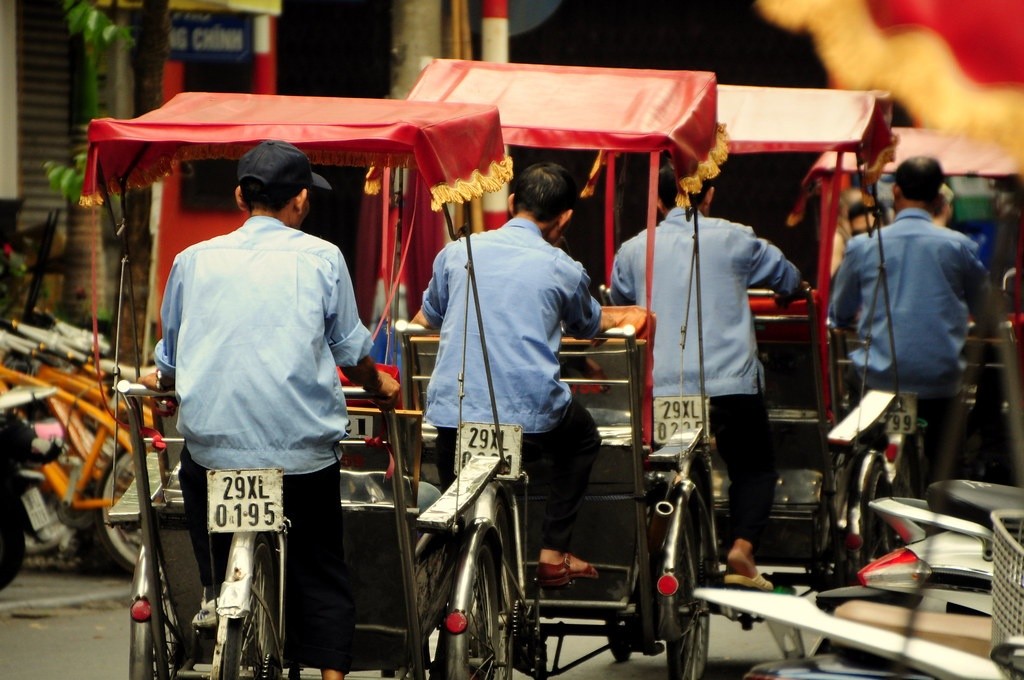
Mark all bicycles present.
[0,310,169,592]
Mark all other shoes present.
[190,586,221,629]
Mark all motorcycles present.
[690,468,1024,680]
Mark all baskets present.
[990,502,1024,654]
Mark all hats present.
[238,139,331,193]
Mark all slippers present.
[724,571,774,594]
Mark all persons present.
[610,161,810,593]
[409,163,619,680]
[152,139,401,680]
[829,156,986,501]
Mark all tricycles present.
[80,56,1019,680]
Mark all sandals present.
[536,552,599,588]
